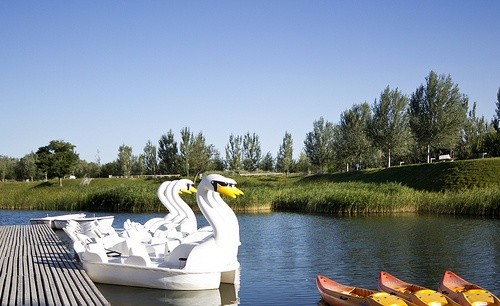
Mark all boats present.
[377,270,462,306]
[53,215,115,229]
[437,269,500,306]
[315,274,418,306]
[62,172,245,291]
[30,212,86,229]
